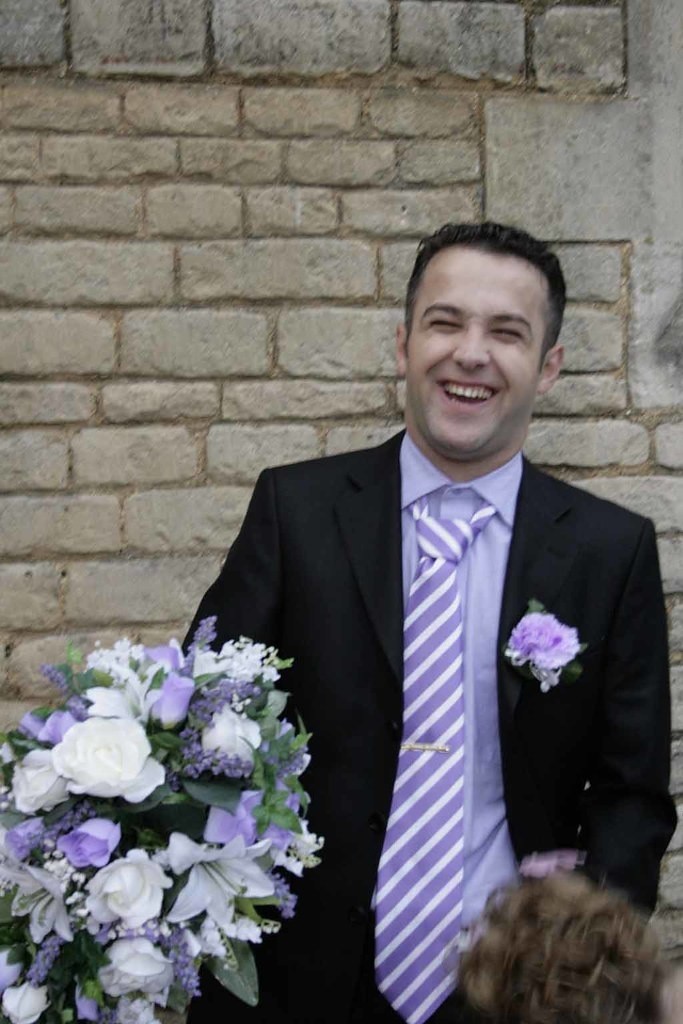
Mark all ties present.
[374,496,500,1023]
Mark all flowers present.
[501,595,589,695]
[0,615,329,1024]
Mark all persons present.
[177,214,677,1024]
